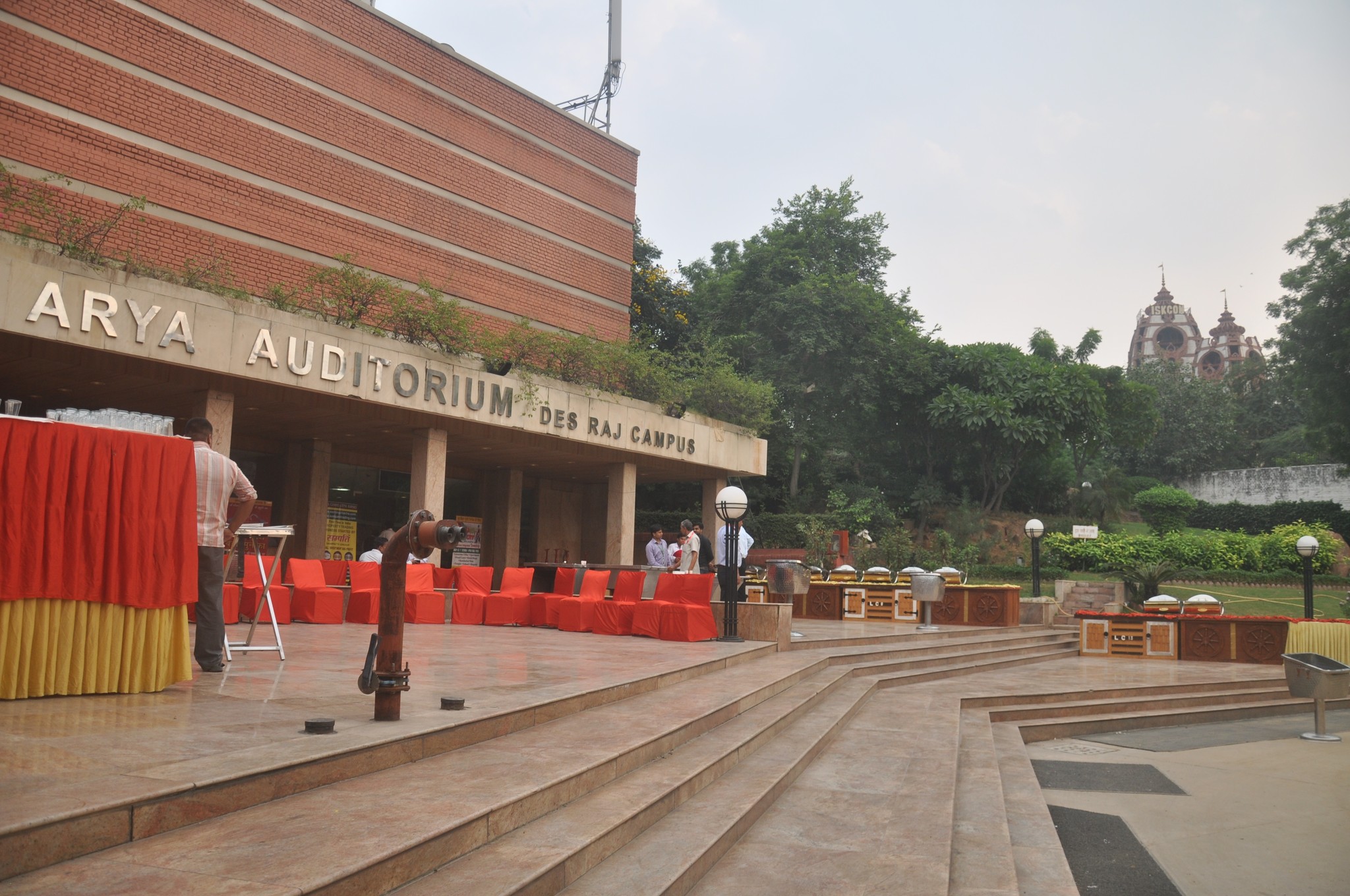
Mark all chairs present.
[213,542,718,640]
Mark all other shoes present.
[205,663,223,672]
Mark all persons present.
[737,526,755,602]
[378,520,396,541]
[357,537,420,565]
[692,522,714,574]
[644,524,673,573]
[182,417,259,673]
[668,519,701,574]
[716,519,743,602]
[667,533,685,571]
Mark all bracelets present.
[228,527,235,535]
[674,565,677,568]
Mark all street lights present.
[1295,536,1320,618]
[713,484,749,641]
[1024,518,1045,597]
